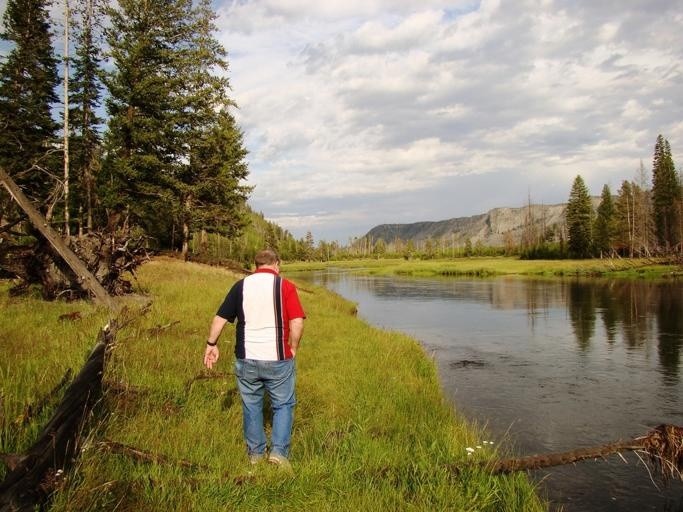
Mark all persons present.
[204,251,306,470]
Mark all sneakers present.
[268,452,293,472]
[250,453,267,464]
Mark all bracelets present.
[207,340,216,346]
